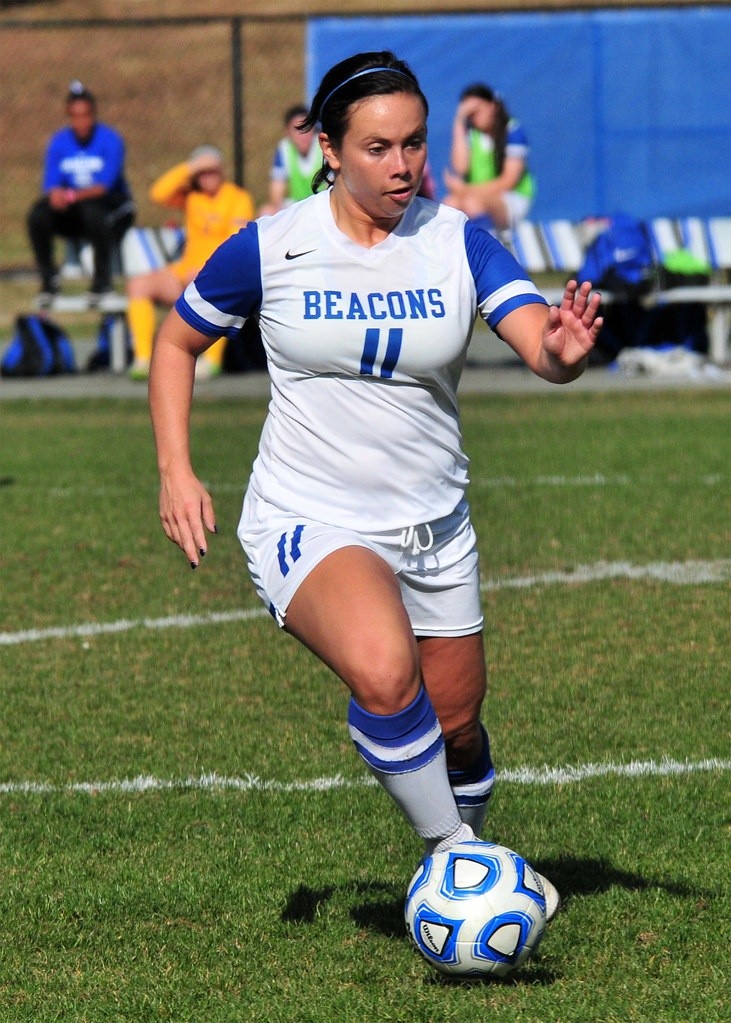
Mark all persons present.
[24,89,137,311]
[148,50,606,861]
[126,142,258,387]
[439,81,536,253]
[258,99,334,220]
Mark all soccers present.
[404,841,547,978]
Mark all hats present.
[67,87,94,104]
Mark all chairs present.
[122,209,731,368]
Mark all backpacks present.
[0,314,77,378]
[86,310,134,377]
[577,213,662,298]
